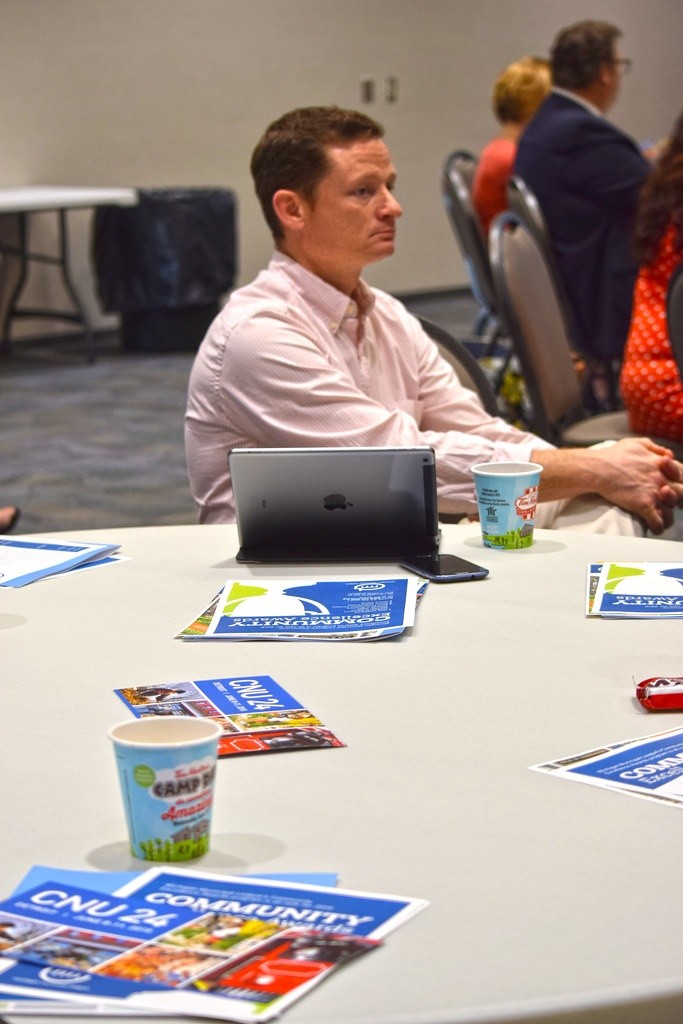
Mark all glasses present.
[607,57,633,76]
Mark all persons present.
[469,57,550,248]
[610,110,683,447]
[513,20,654,360]
[183,105,683,538]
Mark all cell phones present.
[398,554,489,583]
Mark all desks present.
[1,518,682,1023]
[0,181,136,365]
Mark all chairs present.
[413,147,683,465]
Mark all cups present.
[471,461,544,549]
[108,715,223,861]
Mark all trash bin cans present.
[87,185,239,353]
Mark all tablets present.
[225,447,440,562]
